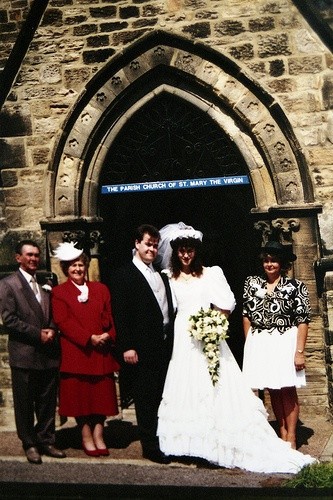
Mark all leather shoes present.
[41,442,65,457]
[26,445,41,463]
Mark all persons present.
[242,240,311,449]
[111,224,174,464]
[50,241,121,457]
[0,240,65,465]
[132,222,320,473]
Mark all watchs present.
[296,349,303,353]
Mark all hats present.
[258,242,297,261]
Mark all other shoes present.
[149,451,171,464]
[169,455,180,463]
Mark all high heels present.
[92,435,109,455]
[81,437,99,456]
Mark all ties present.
[30,277,38,294]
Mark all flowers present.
[188,306,230,386]
[161,269,171,279]
[42,284,52,296]
